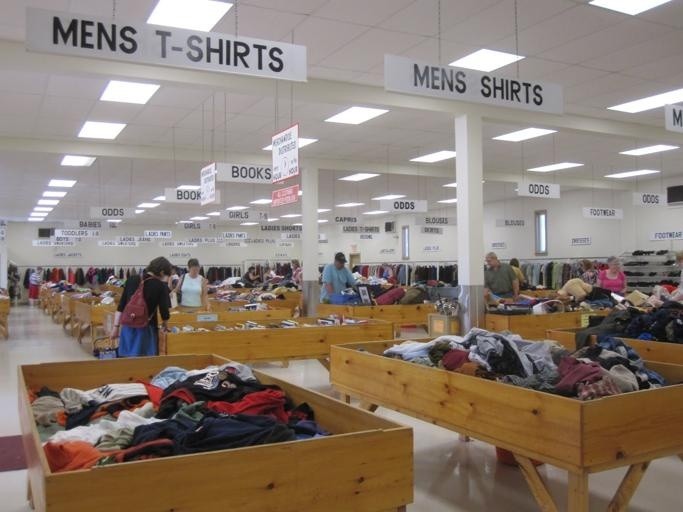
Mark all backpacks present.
[120,274,158,329]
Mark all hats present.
[336,253,346,262]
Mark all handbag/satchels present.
[176,290,181,304]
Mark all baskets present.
[94,336,120,359]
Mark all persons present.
[262,266,272,283]
[290,259,302,283]
[482,252,520,306]
[28,266,42,308]
[578,259,598,284]
[242,265,260,284]
[351,266,362,279]
[509,257,527,291]
[109,255,173,357]
[668,250,682,302]
[168,264,178,290]
[171,258,210,312]
[319,252,355,303]
[381,262,393,280]
[596,256,627,297]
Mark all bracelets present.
[113,325,119,327]
